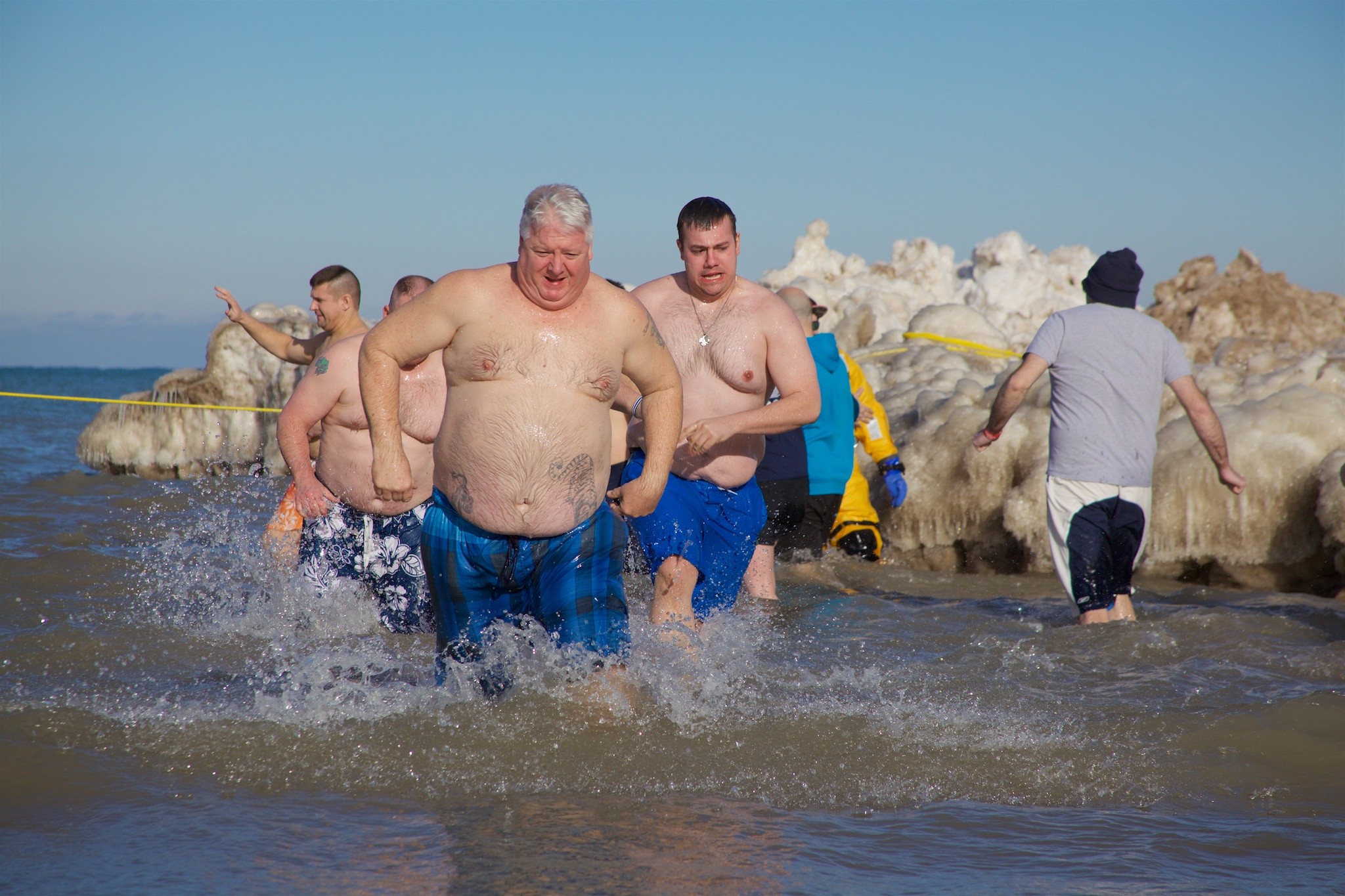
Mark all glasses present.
[812,322,819,330]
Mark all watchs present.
[882,463,906,472]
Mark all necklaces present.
[688,274,738,347]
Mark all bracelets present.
[985,428,1002,441]
[632,396,642,418]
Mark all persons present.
[971,247,1245,626]
[276,274,448,634]
[776,288,854,561]
[758,388,810,564]
[215,265,368,561]
[809,298,907,561]
[359,184,685,696]
[611,196,821,644]
[604,279,631,503]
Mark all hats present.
[809,298,827,317]
[1081,246,1143,308]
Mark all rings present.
[694,424,697,430]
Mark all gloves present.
[878,454,912,507]
